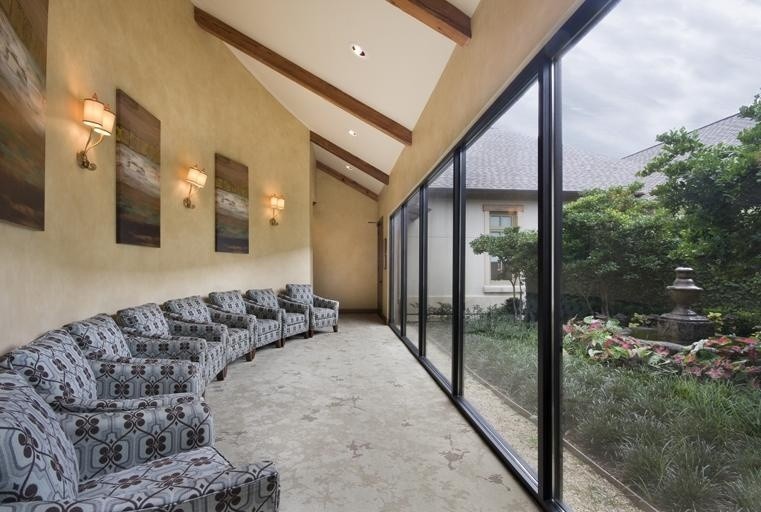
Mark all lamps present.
[78,92,116,171]
[184,164,207,208]
[269,194,285,226]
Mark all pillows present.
[116,303,174,339]
[286,284,314,306]
[247,288,279,308]
[161,295,211,323]
[2,357,82,502]
[2,329,100,398]
[209,290,245,314]
[62,312,130,357]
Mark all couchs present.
[48,357,210,409]
[243,294,310,343]
[204,300,283,358]
[81,337,207,398]
[159,308,253,376]
[1,410,281,511]
[123,320,226,388]
[276,293,338,337]
